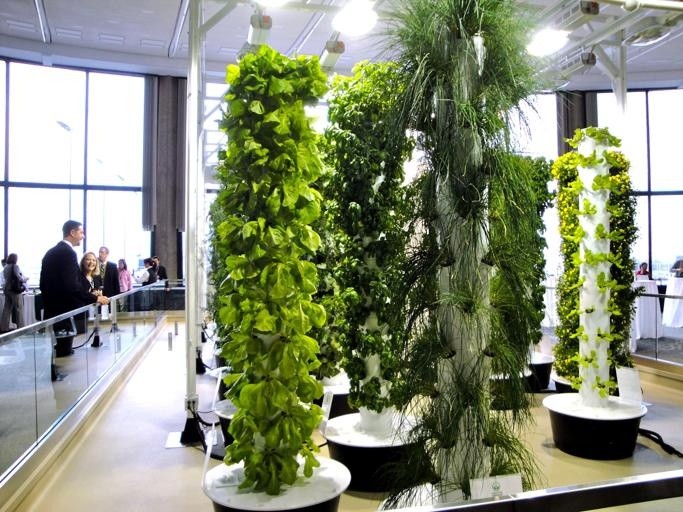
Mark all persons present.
[0,258,17,327]
[40,221,110,381]
[137,258,158,288]
[635,263,650,280]
[116,258,131,295]
[151,256,169,291]
[0,253,28,334]
[79,251,104,305]
[669,259,682,277]
[95,247,119,300]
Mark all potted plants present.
[203,0,647,512]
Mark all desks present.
[633,279,683,339]
[0,290,36,330]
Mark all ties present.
[100,264,105,276]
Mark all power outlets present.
[185,393,199,411]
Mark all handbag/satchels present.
[11,277,26,295]
